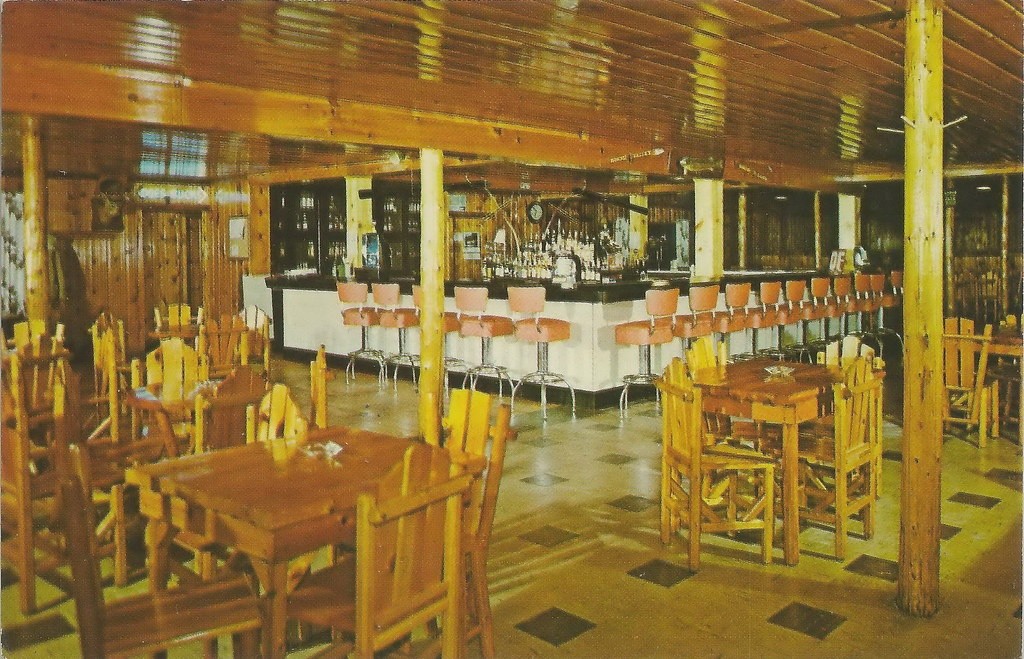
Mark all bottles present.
[325,191,355,279]
[276,187,318,276]
[480,217,647,286]
[376,190,420,278]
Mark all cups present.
[669,260,679,272]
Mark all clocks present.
[527,201,544,225]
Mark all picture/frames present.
[227,216,249,260]
[449,195,467,212]
[464,232,480,260]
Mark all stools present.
[336,268,905,420]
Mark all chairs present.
[1,306,1022,658]
